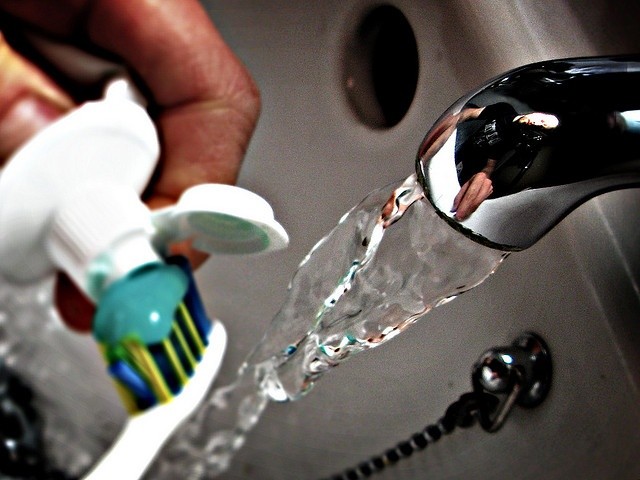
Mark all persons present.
[418,102,564,222]
[0,0,262,331]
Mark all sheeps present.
[415,57,636,250]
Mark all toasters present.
[82,252,227,480]
